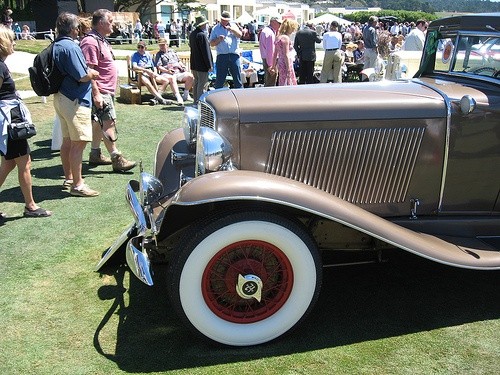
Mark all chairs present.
[206,50,375,87]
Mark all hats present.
[269,16,283,24]
[193,15,208,27]
[158,38,167,45]
[137,41,146,47]
[220,10,231,21]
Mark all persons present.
[49,11,100,197]
[1,9,35,40]
[259,17,282,86]
[293,21,323,84]
[295,19,367,41]
[131,42,173,106]
[104,15,198,49]
[320,21,343,83]
[154,38,194,107]
[340,16,430,81]
[75,16,94,43]
[270,19,299,86]
[188,15,214,105]
[239,18,261,41]
[209,12,243,88]
[78,9,138,171]
[0,26,53,221]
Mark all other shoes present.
[192,103,198,108]
[183,90,188,101]
[69,186,98,197]
[159,98,174,104]
[149,98,158,104]
[23,206,52,217]
[176,98,184,106]
[0,212,6,223]
[61,180,73,192]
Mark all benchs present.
[126,54,195,105]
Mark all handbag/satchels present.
[8,122,36,141]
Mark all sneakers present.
[88,151,112,164]
[112,154,137,172]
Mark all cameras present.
[91,102,113,121]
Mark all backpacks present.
[28,37,74,96]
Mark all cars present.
[108,29,131,45]
[93,13,500,348]
[150,26,166,38]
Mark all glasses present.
[422,24,427,30]
[138,46,144,49]
[71,24,81,30]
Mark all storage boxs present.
[119,83,138,102]
[129,87,140,104]
